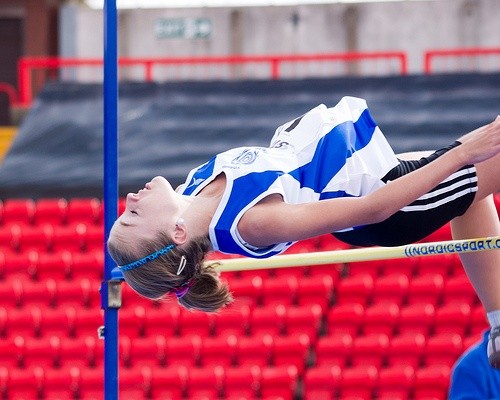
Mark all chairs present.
[0,195,499,400]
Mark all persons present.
[107,95,500,370]
